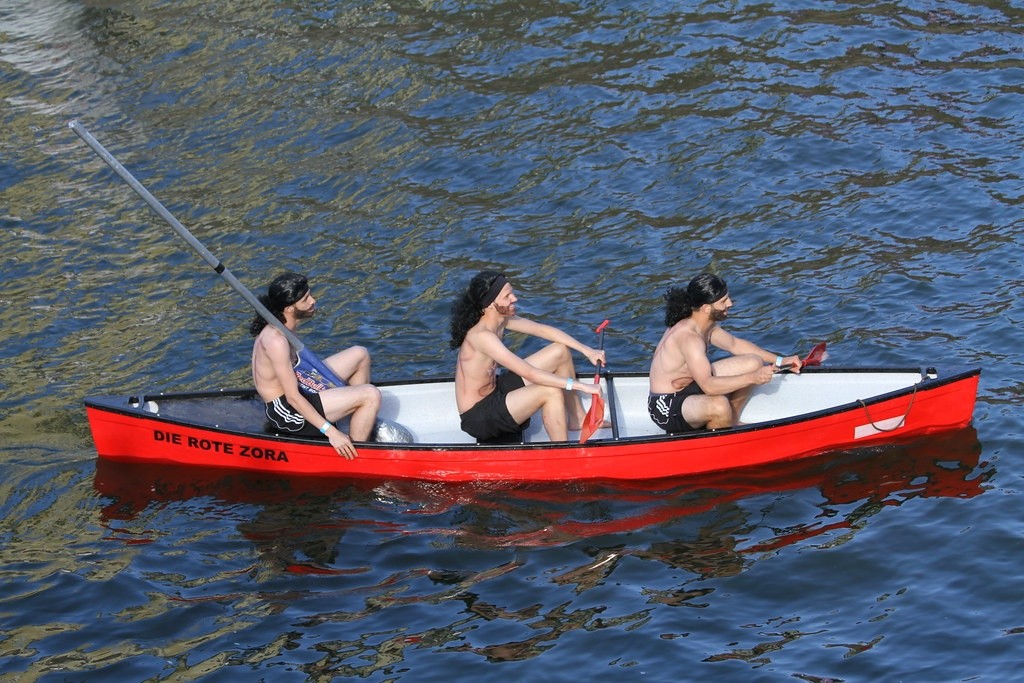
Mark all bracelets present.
[566,378,573,390]
[320,422,331,434]
[776,356,783,366]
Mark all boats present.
[84,362,985,480]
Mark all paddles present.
[773,342,826,374]
[580,320,610,444]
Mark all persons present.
[647,273,802,431]
[250,274,382,461]
[450,270,612,443]
